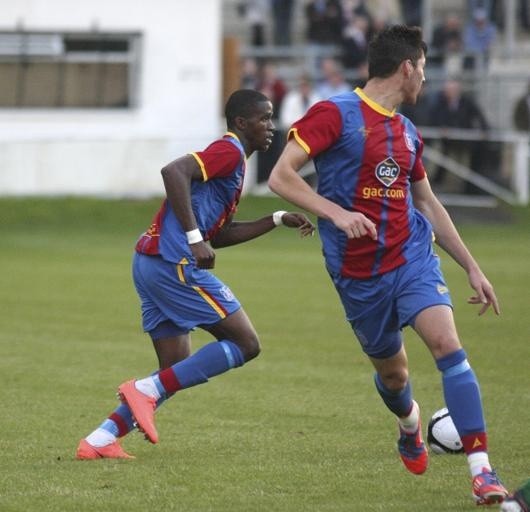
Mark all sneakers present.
[119,379,159,444]
[395,401,429,474]
[470,467,508,505]
[76,436,136,459]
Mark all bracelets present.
[184,229,205,247]
[273,210,287,229]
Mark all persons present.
[71,89,317,461]
[219,0,530,206]
[495,473,530,510]
[263,23,516,507]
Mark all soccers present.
[426,407,465,454]
